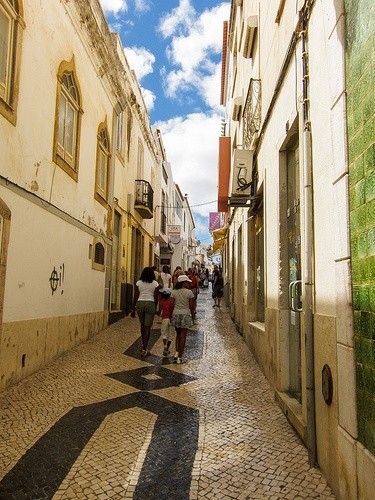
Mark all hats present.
[163,288,171,294]
[177,275,192,283]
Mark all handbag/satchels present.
[155,273,164,290]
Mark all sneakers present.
[173,351,179,360]
[177,357,187,365]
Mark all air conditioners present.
[225,149,256,205]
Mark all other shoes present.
[167,351,172,355]
[213,304,217,308]
[217,305,220,308]
[141,349,152,356]
[162,345,167,357]
